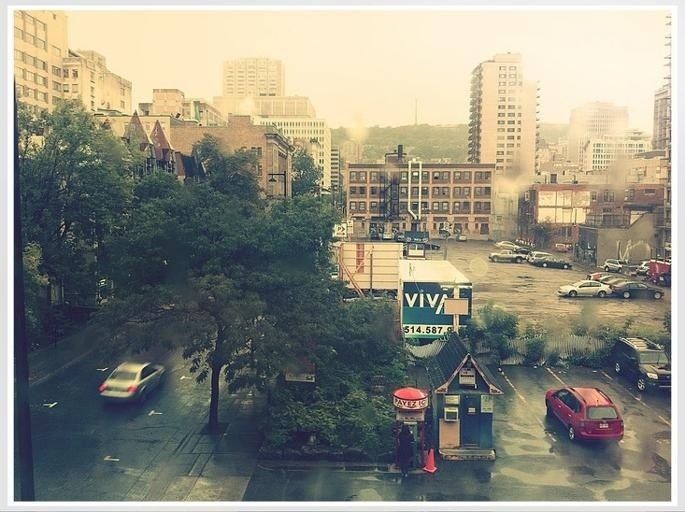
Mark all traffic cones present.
[422,447,438,473]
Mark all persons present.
[394,423,415,479]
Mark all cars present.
[456,233,467,242]
[369,230,431,242]
[97,359,166,404]
[544,384,624,441]
[611,335,671,392]
[556,257,665,299]
[490,241,571,269]
